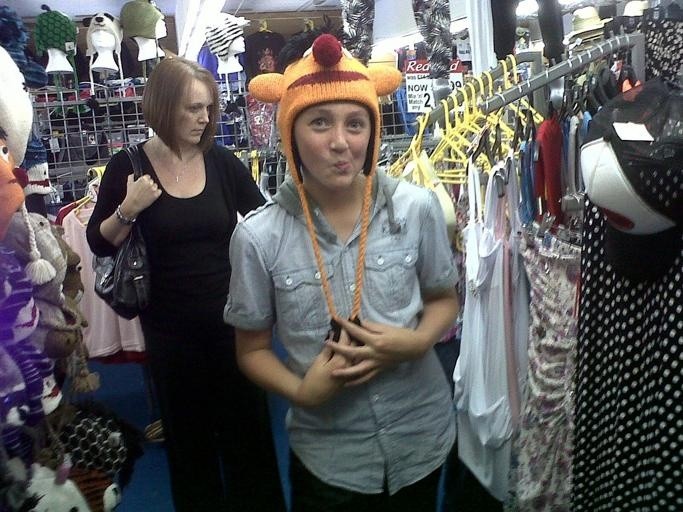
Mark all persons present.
[219,36,459,509]
[85,58,268,509]
[119,0,166,62]
[204,12,246,74]
[88,15,119,74]
[36,12,77,74]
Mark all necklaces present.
[152,140,192,183]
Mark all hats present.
[0,46,33,166]
[577,74,682,285]
[81,11,124,56]
[246,31,403,187]
[119,0,163,48]
[0,212,99,415]
[0,5,29,65]
[0,403,164,512]
[203,11,251,63]
[31,3,77,58]
[18,132,54,198]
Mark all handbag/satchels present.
[89,144,152,322]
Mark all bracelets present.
[114,204,136,228]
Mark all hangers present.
[254,15,316,35]
[377,0,682,254]
[74,168,105,226]
[233,148,295,205]
